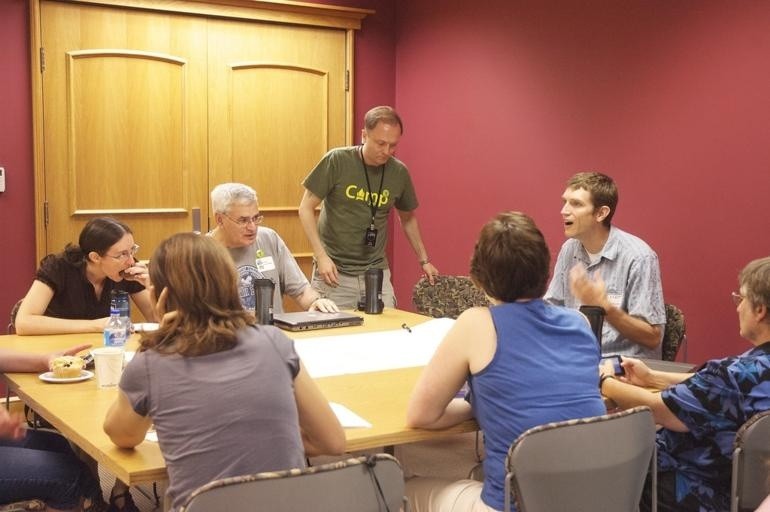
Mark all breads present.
[76,347,94,364]
[51,356,86,378]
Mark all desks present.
[0,303,581,489]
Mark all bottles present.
[104,310,128,348]
[253,278,276,326]
[361,267,385,314]
[110,289,130,316]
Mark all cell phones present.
[598,353,626,378]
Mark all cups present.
[579,304,607,354]
[91,348,126,387]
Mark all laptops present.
[273,311,366,332]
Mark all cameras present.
[363,227,379,247]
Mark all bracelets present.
[600,372,615,395]
[419,257,431,265]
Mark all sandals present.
[109,487,141,512]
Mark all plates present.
[39,370,93,384]
[133,320,159,334]
[127,349,134,359]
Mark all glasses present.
[732,291,749,303]
[222,211,263,226]
[101,245,138,262]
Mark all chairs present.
[408,275,492,317]
[654,296,689,363]
[184,451,415,512]
[675,408,770,510]
[504,404,660,511]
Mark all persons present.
[103,232,346,512]
[599,257,770,512]
[15,217,159,512]
[0,343,94,512]
[544,171,667,360]
[403,211,607,512]
[206,183,341,315]
[298,106,439,309]
